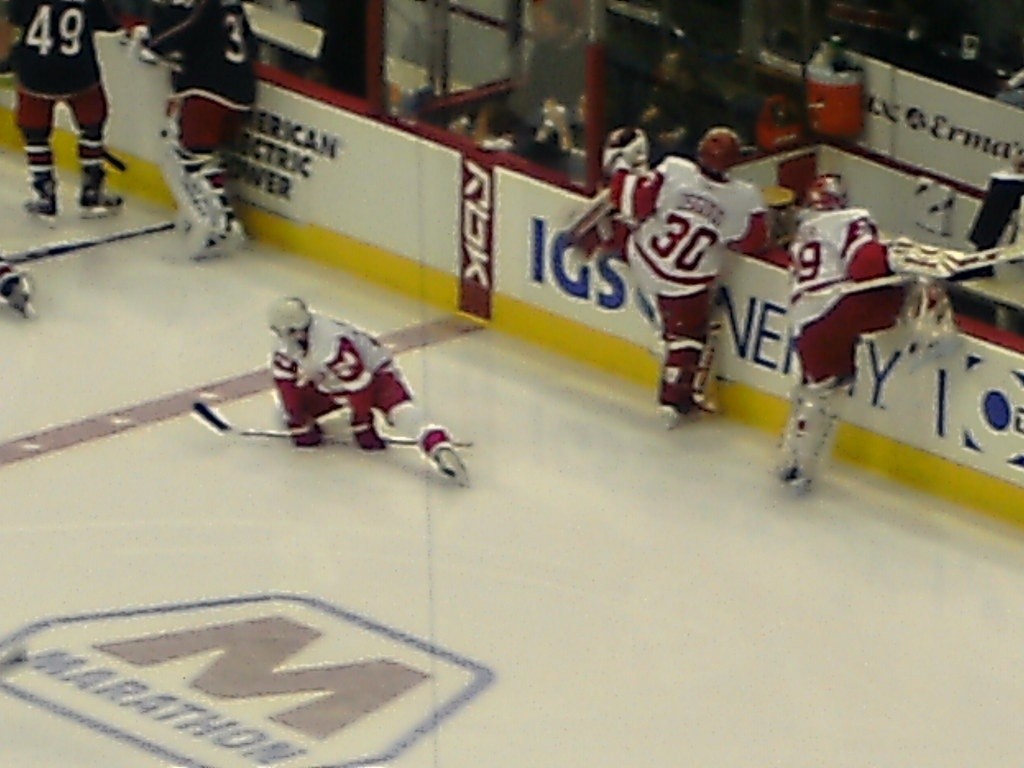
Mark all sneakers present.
[76,172,124,218]
[23,178,59,222]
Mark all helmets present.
[804,176,849,212]
[265,297,311,334]
[697,128,746,175]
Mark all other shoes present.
[0,260,34,319]
[417,425,472,487]
[196,221,245,263]
[787,470,812,495]
[660,399,692,432]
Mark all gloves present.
[288,417,323,448]
[349,414,387,451]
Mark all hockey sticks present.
[102,148,128,173]
[0,221,176,265]
[189,399,474,449]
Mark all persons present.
[563,123,770,431]
[609,48,731,181]
[948,152,1024,282]
[470,0,632,171]
[0,0,260,261]
[995,68,1024,111]
[267,296,469,488]
[773,174,958,504]
